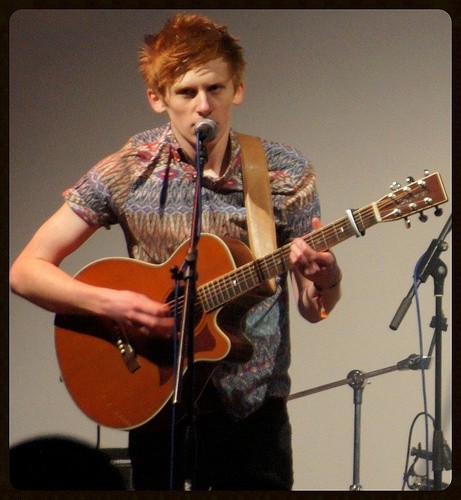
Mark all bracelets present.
[313,277,341,318]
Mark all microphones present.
[194,118,218,141]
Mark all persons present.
[9,15,342,491]
[9,435,123,491]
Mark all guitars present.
[53,167,449,433]
